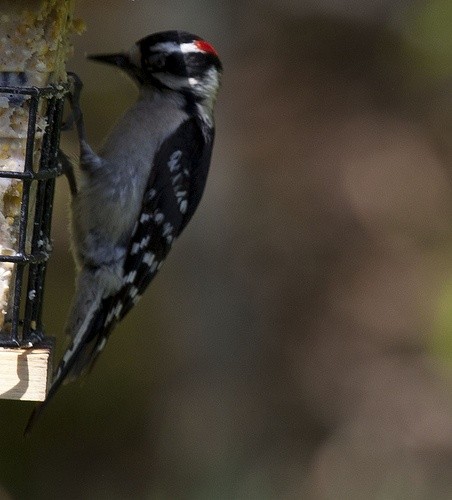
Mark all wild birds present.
[21,29,222,440]
